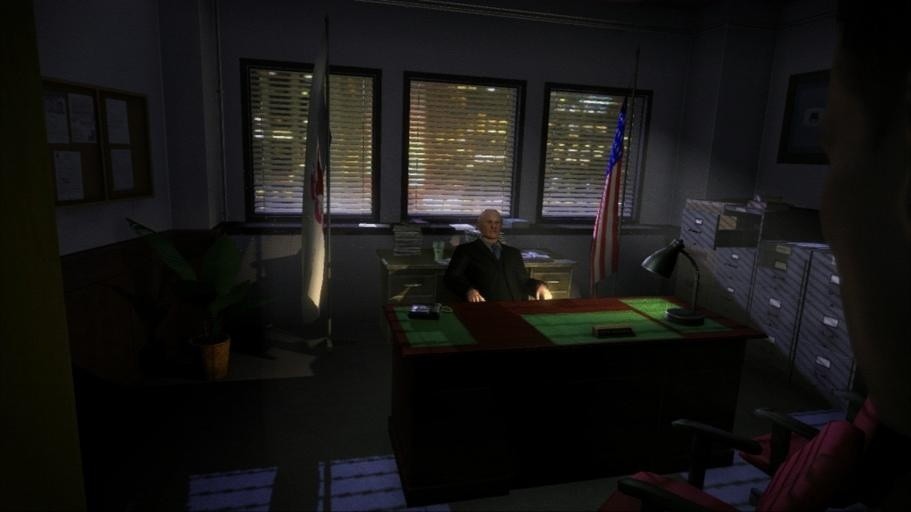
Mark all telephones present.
[408,303,442,320]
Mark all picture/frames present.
[777,69,829,165]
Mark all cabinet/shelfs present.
[40,78,153,208]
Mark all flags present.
[589,91,628,299]
[301,18,331,325]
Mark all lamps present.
[642,236,704,324]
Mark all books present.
[393,223,423,257]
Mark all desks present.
[375,247,580,345]
[381,294,742,508]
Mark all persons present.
[820,0,911,439]
[444,210,553,304]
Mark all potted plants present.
[122,215,274,380]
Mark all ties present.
[491,242,501,260]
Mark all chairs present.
[597,391,884,511]
